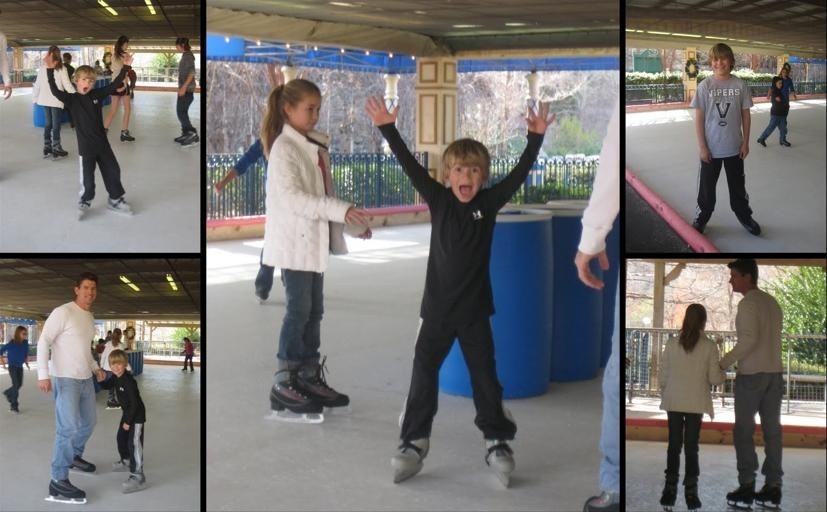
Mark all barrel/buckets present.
[439,199,620,401]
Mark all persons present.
[181,337,194,371]
[574,100,620,511]
[0,31,135,210]
[0,272,148,499]
[363,95,556,474]
[174,37,199,146]
[757,76,791,146]
[213,138,286,300]
[657,304,727,509]
[767,68,796,134]
[260,78,372,414]
[692,43,761,235]
[718,258,784,504]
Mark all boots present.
[2,388,20,412]
[735,211,761,236]
[171,126,199,146]
[266,367,326,416]
[109,459,130,471]
[104,398,122,408]
[120,128,136,142]
[47,478,85,500]
[41,140,69,157]
[657,481,784,509]
[689,207,713,233]
[76,196,132,217]
[120,472,149,489]
[68,455,98,473]
[294,362,351,408]
[484,440,518,473]
[388,436,431,470]
[756,136,792,146]
